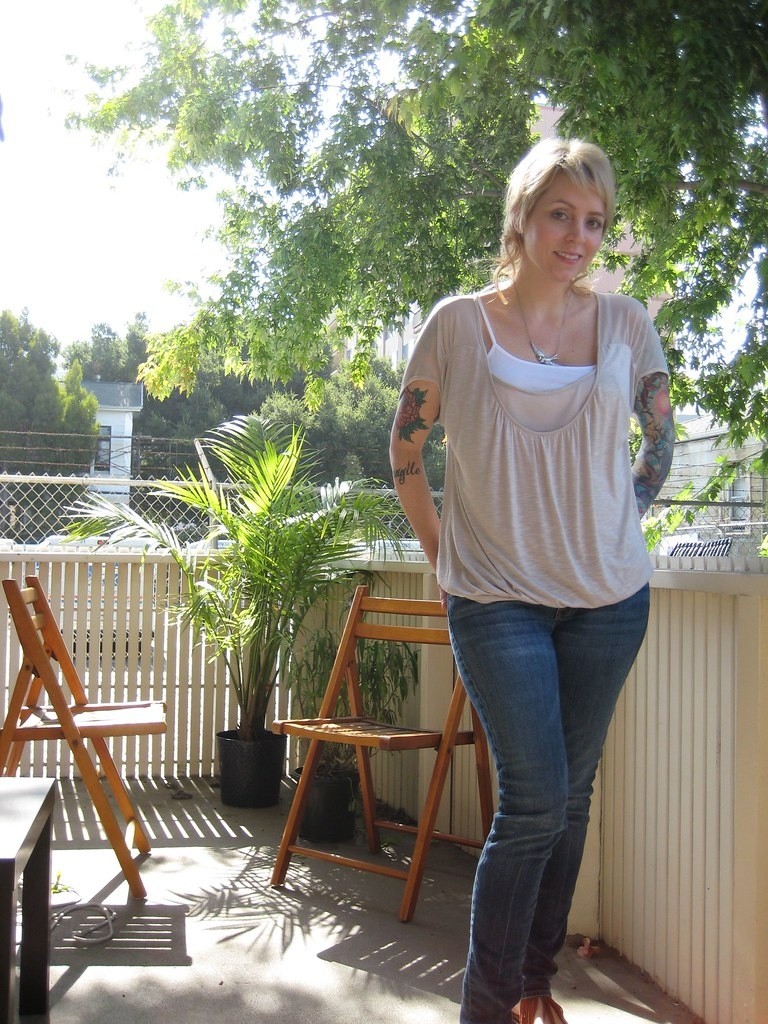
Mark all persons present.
[389,137,675,1024]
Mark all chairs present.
[0,575,168,899]
[268,584,495,924]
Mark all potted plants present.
[285,627,422,843]
[88,414,413,810]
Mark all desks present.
[0,776,56,1024]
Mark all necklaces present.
[512,279,572,365]
